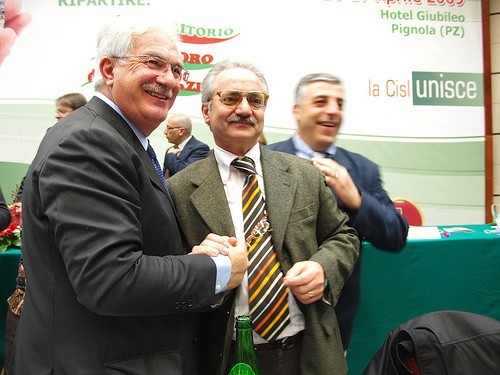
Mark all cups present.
[491,205,500,227]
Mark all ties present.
[230,156,291,344]
[311,152,327,158]
[147,143,172,202]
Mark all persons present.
[0,182,12,365]
[0,0,32,66]
[166,62,347,374]
[163,115,211,180]
[17,16,249,375]
[266,73,408,349]
[13,93,88,215]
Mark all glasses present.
[167,125,181,131]
[113,55,191,82]
[211,90,269,109]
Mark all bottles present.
[227,315,263,375]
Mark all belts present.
[230,329,303,351]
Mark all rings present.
[333,170,338,179]
[309,291,313,298]
